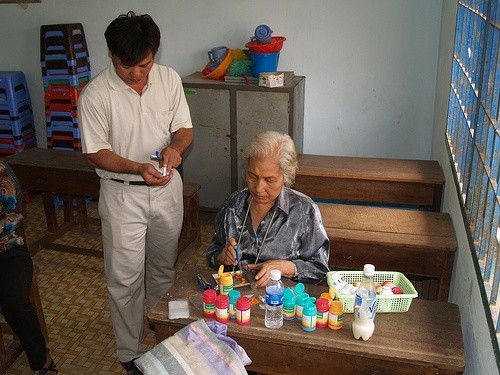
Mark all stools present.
[40,23,95,210]
[0,71,38,203]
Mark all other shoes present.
[123,357,145,375]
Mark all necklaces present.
[232,195,277,270]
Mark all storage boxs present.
[259,72,284,88]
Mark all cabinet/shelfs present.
[177,72,306,212]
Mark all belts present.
[111,179,151,186]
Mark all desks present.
[6,147,201,266]
[147,267,465,375]
[314,203,458,302]
[291,153,445,211]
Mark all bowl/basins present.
[245,36,286,53]
[201,48,234,79]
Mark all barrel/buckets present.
[249,50,280,78]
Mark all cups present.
[208,46,226,61]
[250,25,273,45]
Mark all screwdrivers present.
[235,243,242,278]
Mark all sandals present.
[38,348,58,375]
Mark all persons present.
[207,131,330,287]
[77,11,194,375]
[0,159,58,375]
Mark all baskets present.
[326,270,417,313]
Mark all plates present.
[212,270,250,288]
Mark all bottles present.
[218,265,235,295]
[282,281,344,332]
[203,289,217,318]
[265,269,283,328]
[236,297,250,325]
[216,294,229,323]
[228,289,241,320]
[352,264,377,341]
